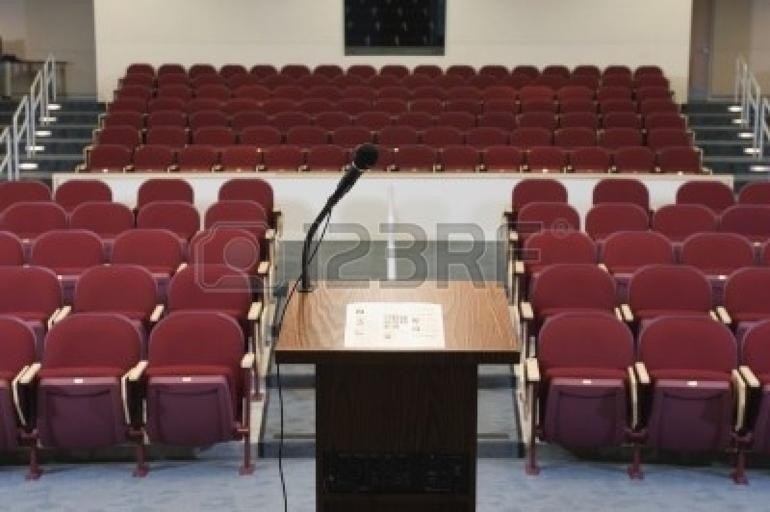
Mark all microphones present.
[326,142,378,206]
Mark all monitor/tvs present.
[344,0,446,58]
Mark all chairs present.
[623,263,717,316]
[736,317,770,484]
[1,178,276,268]
[162,261,263,399]
[1,266,55,328]
[521,262,624,420]
[524,312,639,477]
[35,310,139,477]
[638,315,736,481]
[717,263,770,323]
[0,309,35,477]
[512,178,770,259]
[140,310,254,476]
[56,263,162,329]
